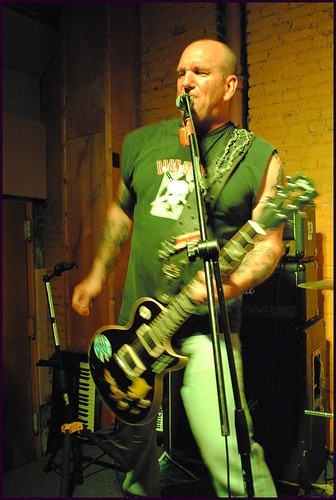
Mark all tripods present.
[42,259,132,496]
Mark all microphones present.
[176,94,194,111]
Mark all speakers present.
[163,316,329,494]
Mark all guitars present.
[88,173,318,426]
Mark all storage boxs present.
[243,203,319,322]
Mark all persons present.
[72,39,284,500]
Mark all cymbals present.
[297,278,333,291]
